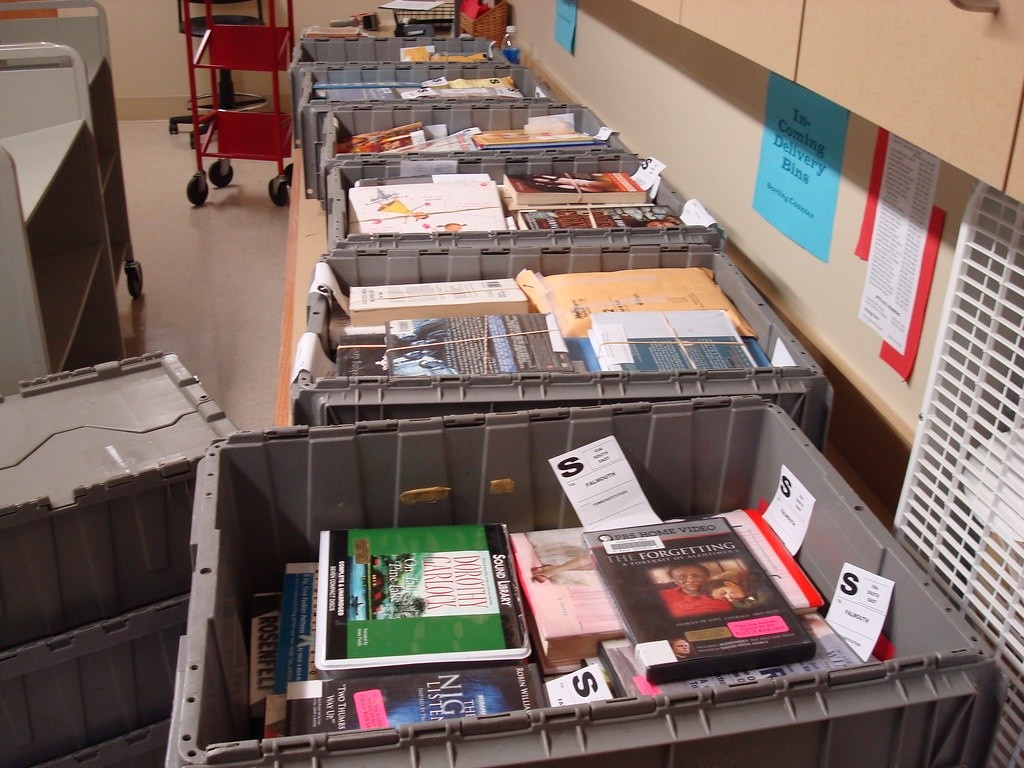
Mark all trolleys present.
[168,1,302,206]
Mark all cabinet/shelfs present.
[0,1,143,399]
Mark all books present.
[312,77,525,101]
[337,120,608,152]
[247,511,885,738]
[336,269,772,379]
[348,170,686,236]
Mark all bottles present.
[502,25,523,65]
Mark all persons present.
[532,555,596,583]
[622,215,677,227]
[700,576,784,613]
[671,639,690,660]
[659,563,733,618]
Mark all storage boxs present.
[0,26,1008,768]
